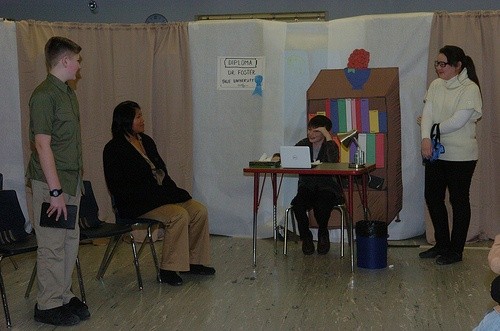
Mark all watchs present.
[50,188,63,197]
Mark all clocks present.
[145,14,168,24]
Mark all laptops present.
[279,146,316,169]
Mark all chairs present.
[25,180,143,303]
[97,179,165,284]
[283,175,351,256]
[0,190,72,328]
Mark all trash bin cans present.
[355,220,388,269]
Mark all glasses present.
[434,60,449,67]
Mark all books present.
[40,202,77,229]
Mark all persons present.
[27,36,91,325]
[272,115,341,255]
[102,100,216,286]
[417,45,482,264]
[473,234,500,331]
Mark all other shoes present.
[34,297,90,326]
[186,263,216,275]
[160,268,183,286]
[419,240,463,265]
[301,229,330,255]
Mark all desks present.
[243,163,375,273]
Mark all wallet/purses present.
[39,202,77,229]
